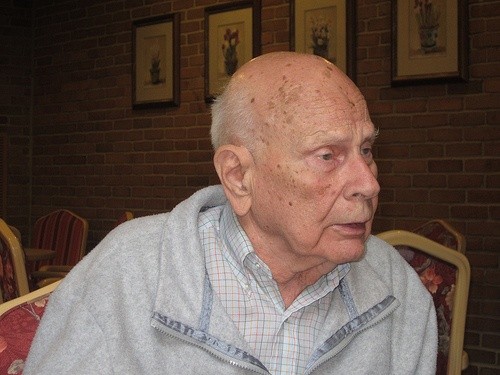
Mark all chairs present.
[374,218,472,375]
[0,209,134,375]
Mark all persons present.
[21,52,438,374]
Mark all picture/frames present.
[390,0,467,86]
[131,12,180,108]
[289,0,358,88]
[205,0,261,103]
